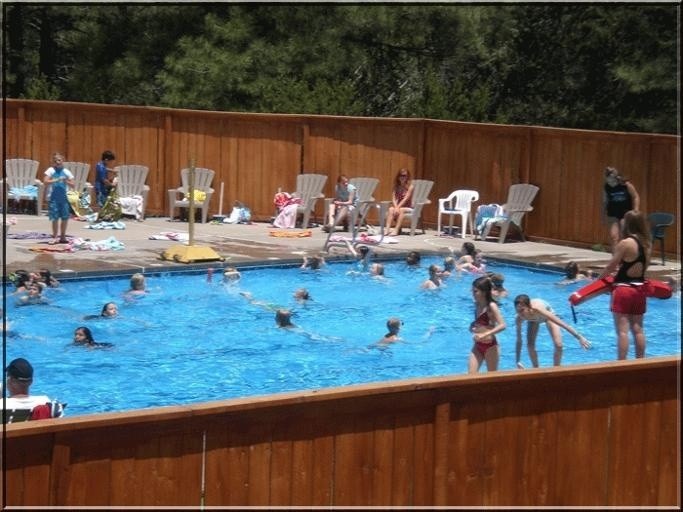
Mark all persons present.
[381,167,415,237]
[73,273,146,344]
[12,269,60,301]
[92,148,120,219]
[597,209,654,360]
[1,358,64,425]
[206,264,241,280]
[600,165,641,262]
[274,242,594,373]
[322,173,360,234]
[41,152,79,238]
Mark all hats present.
[3,357,32,383]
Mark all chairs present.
[435,189,482,238]
[166,165,218,222]
[6,157,150,219]
[634,211,675,267]
[269,172,436,236]
[478,182,544,243]
